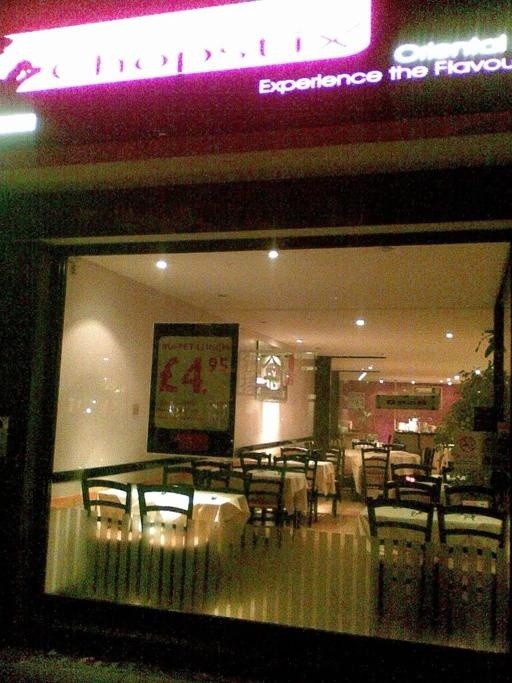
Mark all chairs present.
[345,434,512,641]
